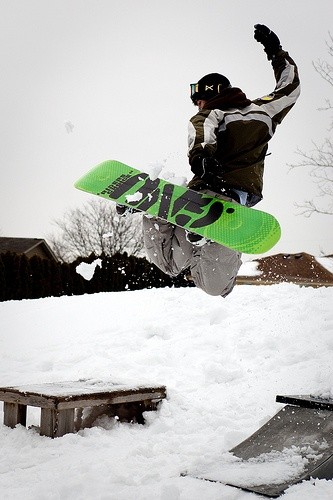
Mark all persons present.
[115,24,302,298]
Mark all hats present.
[192,73,231,101]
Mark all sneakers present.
[183,230,208,247]
[117,204,141,219]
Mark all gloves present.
[254,24,282,60]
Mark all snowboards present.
[74,158,282,255]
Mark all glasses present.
[189,83,226,96]
